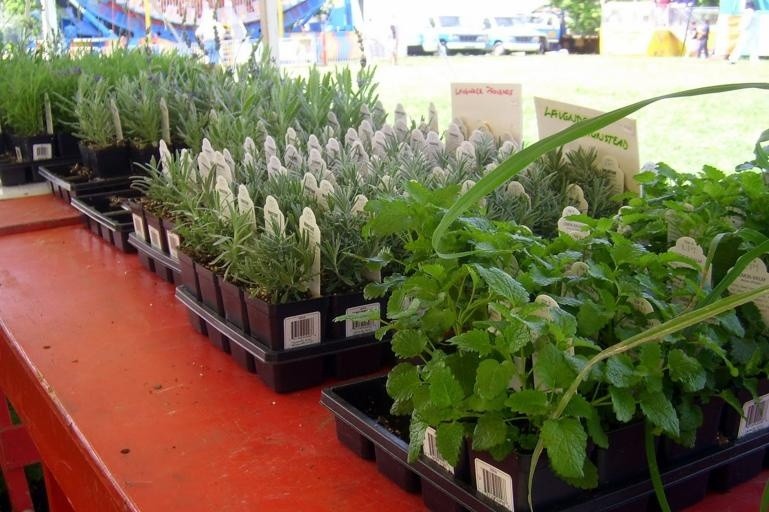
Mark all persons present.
[728,0,761,64]
[689,19,699,55]
[420,16,442,55]
[698,20,710,57]
[194,10,228,62]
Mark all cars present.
[404,9,565,57]
